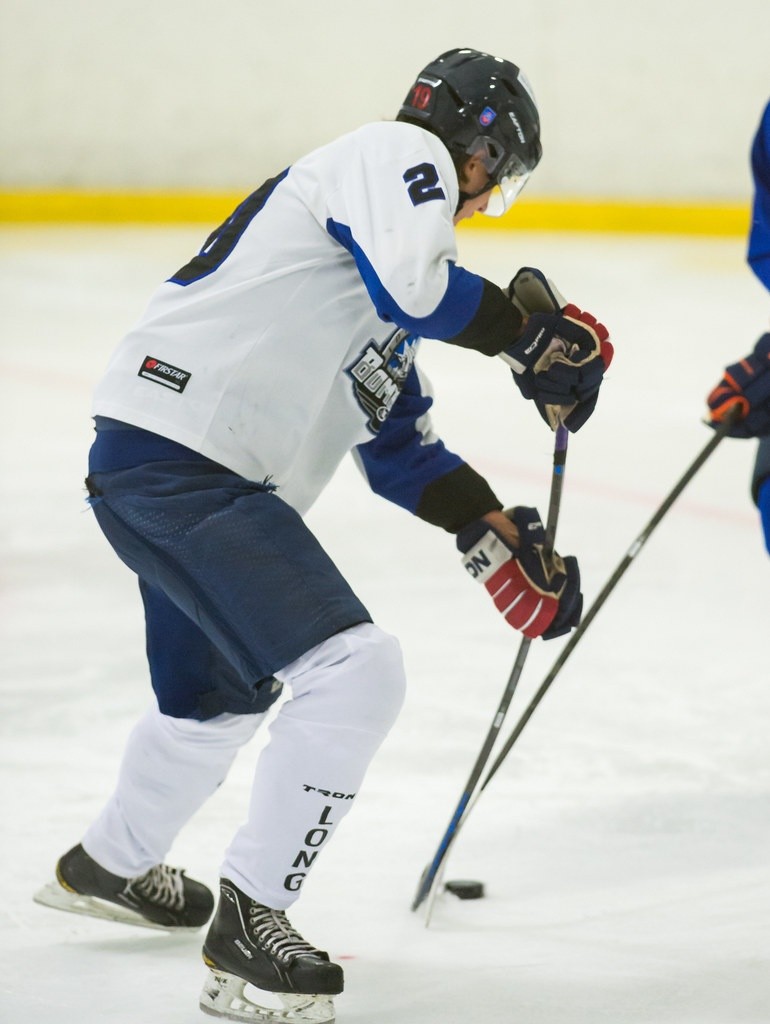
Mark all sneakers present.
[34,843,215,933]
[200,877,344,1024]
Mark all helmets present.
[395,48,542,183]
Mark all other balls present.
[444,880,485,901]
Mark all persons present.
[34,44,613,1024]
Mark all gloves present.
[457,506,583,640]
[498,267,613,431]
[707,332,770,438]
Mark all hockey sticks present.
[405,419,572,909]
[413,407,746,927]
